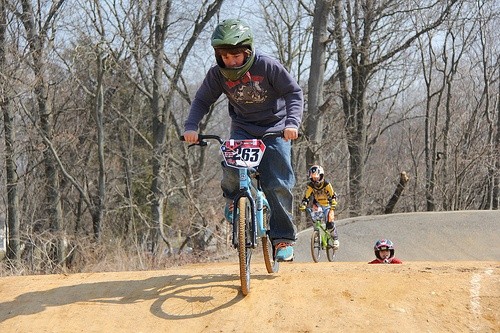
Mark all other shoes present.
[332,239,339,249]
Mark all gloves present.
[331,201,337,210]
[302,197,309,206]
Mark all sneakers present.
[224,199,234,225]
[275,242,293,260]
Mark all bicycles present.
[298,203,338,263]
[179,129,304,296]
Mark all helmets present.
[211,18,256,82]
[373,239,396,263]
[308,165,324,185]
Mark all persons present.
[367,239,402,264]
[299,164,340,249]
[183,19,304,262]
